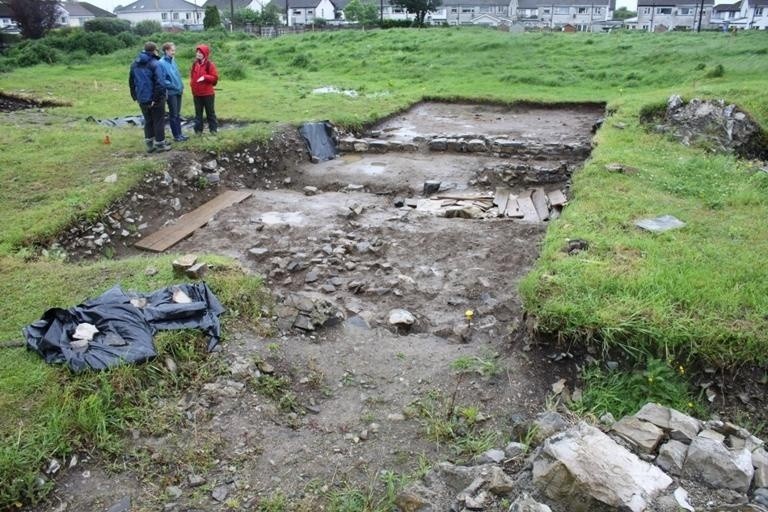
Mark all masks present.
[196,53,204,60]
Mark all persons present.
[154,49,172,152]
[189,45,220,137]
[158,42,190,143]
[128,42,165,155]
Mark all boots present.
[145,138,171,152]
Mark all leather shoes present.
[176,135,188,141]
